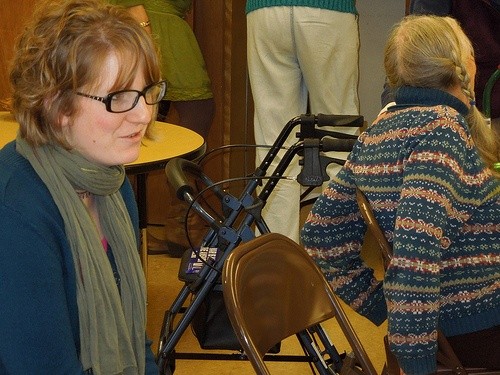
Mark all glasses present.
[72,78,168,113]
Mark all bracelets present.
[138,19,150,27]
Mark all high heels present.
[139,230,167,255]
[164,198,209,258]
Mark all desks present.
[1,109,209,306]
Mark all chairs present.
[355,187,489,375]
[225,228,380,375]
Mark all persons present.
[302,13,499,374]
[109,0,219,257]
[244,0,363,244]
[0,0,171,375]
